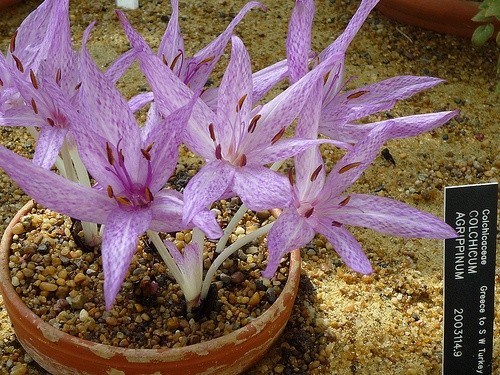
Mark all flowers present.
[1,0,458,316]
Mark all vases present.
[372,0,500,43]
[1,163,301,374]
